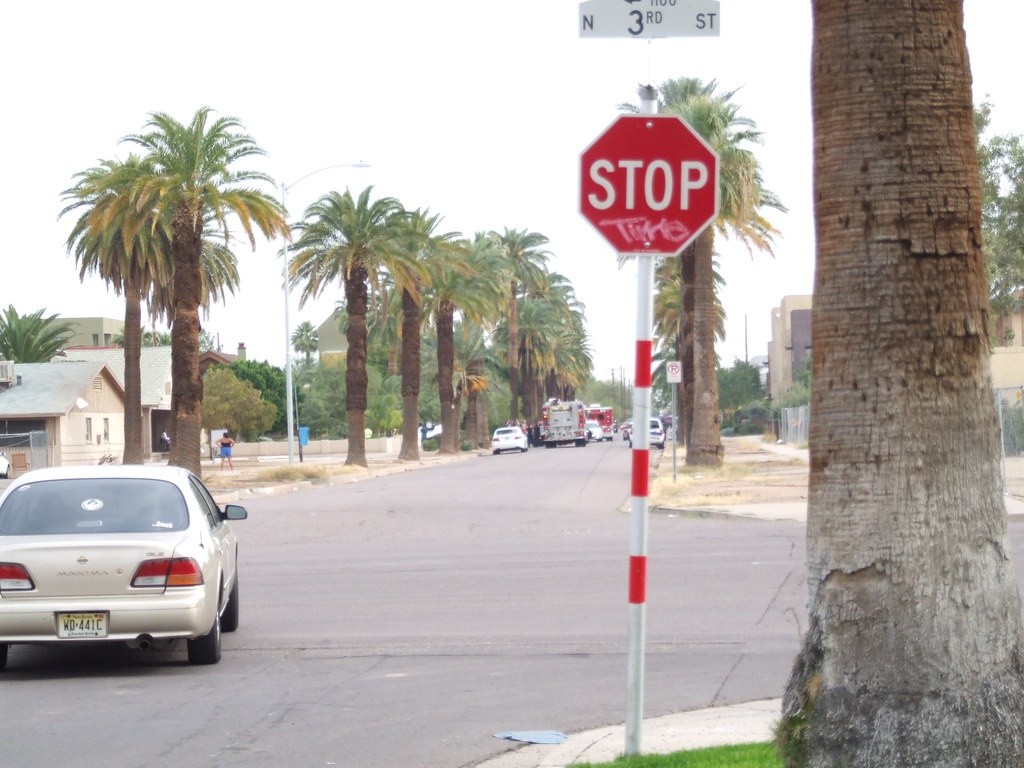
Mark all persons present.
[215,433,235,471]
[160,427,170,451]
[505,417,540,447]
[417,423,428,443]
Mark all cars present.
[623,416,665,451]
[0,464,248,673]
[0,452,11,478]
[492,427,529,455]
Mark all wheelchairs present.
[158,437,170,452]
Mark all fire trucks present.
[583,404,615,442]
[542,398,586,448]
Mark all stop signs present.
[578,111,723,257]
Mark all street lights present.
[281,163,372,462]
